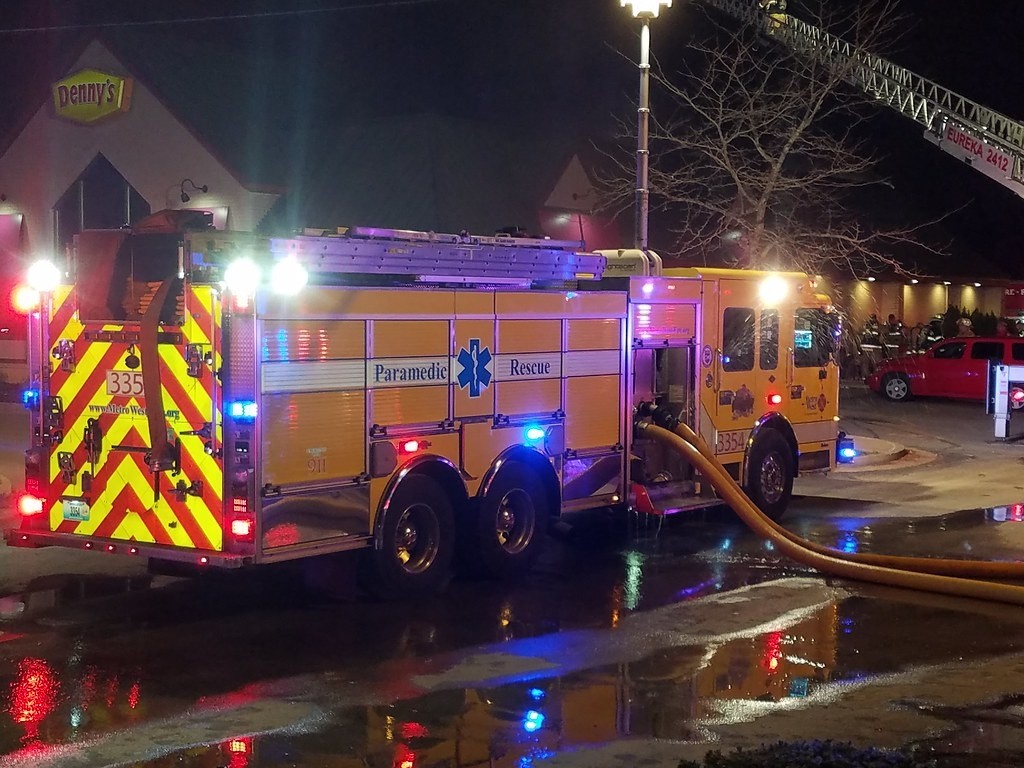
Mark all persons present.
[1015,319,1024,339]
[957,317,976,337]
[864,313,881,344]
[910,322,924,350]
[888,314,906,336]
[997,321,1012,337]
[918,319,944,350]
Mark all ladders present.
[204,225,607,282]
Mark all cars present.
[863,338,1024,413]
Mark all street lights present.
[620,0,674,256]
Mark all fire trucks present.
[3,210,859,603]
